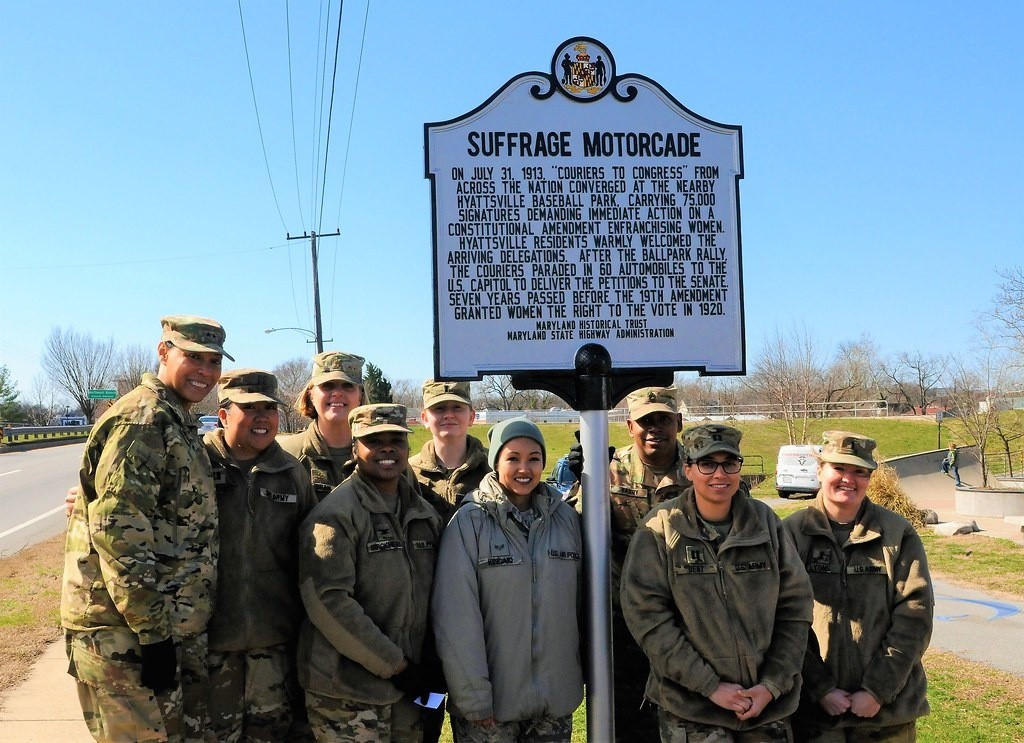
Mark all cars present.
[197,416,219,436]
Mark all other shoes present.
[956,482,960,486]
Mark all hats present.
[487,417,546,473]
[217,369,289,408]
[161,316,236,361]
[422,379,471,409]
[311,352,362,385]
[626,385,678,420]
[349,403,414,442]
[821,431,876,469]
[682,424,741,461]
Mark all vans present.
[775,444,823,498]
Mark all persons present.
[781,431,935,743]
[565,385,751,743]
[432,417,584,743]
[941,442,961,487]
[281,352,366,503]
[298,402,443,743]
[620,423,814,743]
[408,377,492,523]
[59,315,235,743]
[66,370,318,743]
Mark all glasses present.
[690,457,744,475]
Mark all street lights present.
[264,327,316,355]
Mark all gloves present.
[391,660,435,708]
[140,635,177,691]
[569,430,615,485]
